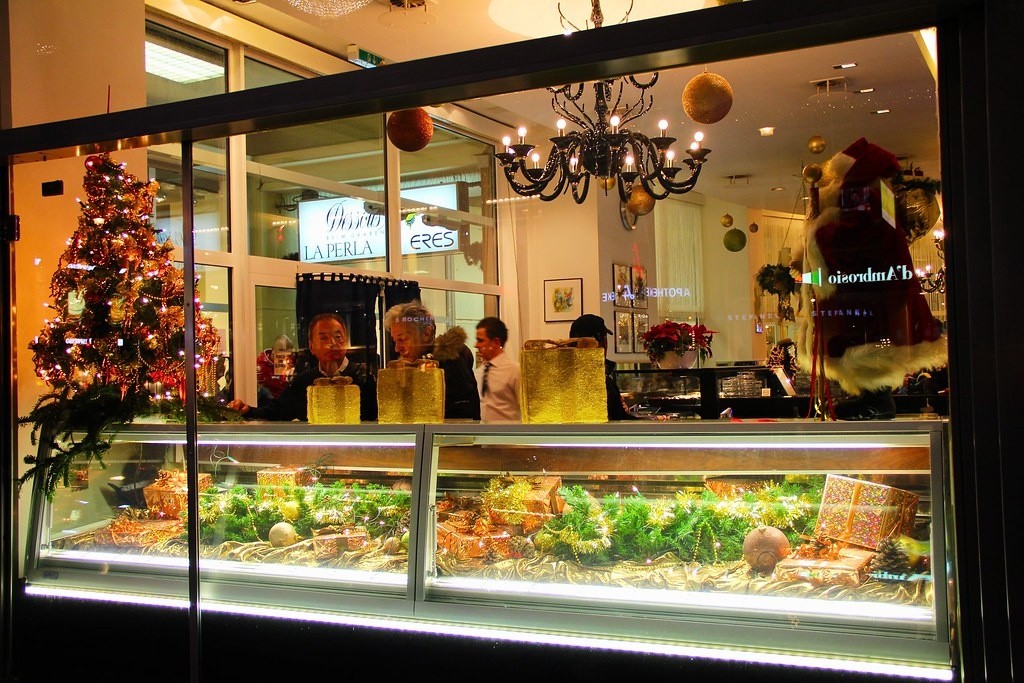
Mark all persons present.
[256,335,295,399]
[226,313,378,421]
[385,299,481,421]
[570,314,641,420]
[473,317,521,421]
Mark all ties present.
[482,363,493,397]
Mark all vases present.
[656,351,698,369]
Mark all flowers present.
[641,320,718,366]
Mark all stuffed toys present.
[790,136,949,421]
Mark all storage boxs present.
[376,367,445,422]
[312,525,369,558]
[437,476,563,558]
[94,471,212,547]
[306,385,360,424]
[776,474,921,585]
[519,348,609,424]
[256,466,313,502]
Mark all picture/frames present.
[632,313,649,354]
[543,278,583,322]
[613,264,631,308]
[631,267,648,309]
[614,311,634,353]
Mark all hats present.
[571,314,613,345]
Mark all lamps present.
[759,127,775,136]
[915,230,945,296]
[494,0,711,204]
